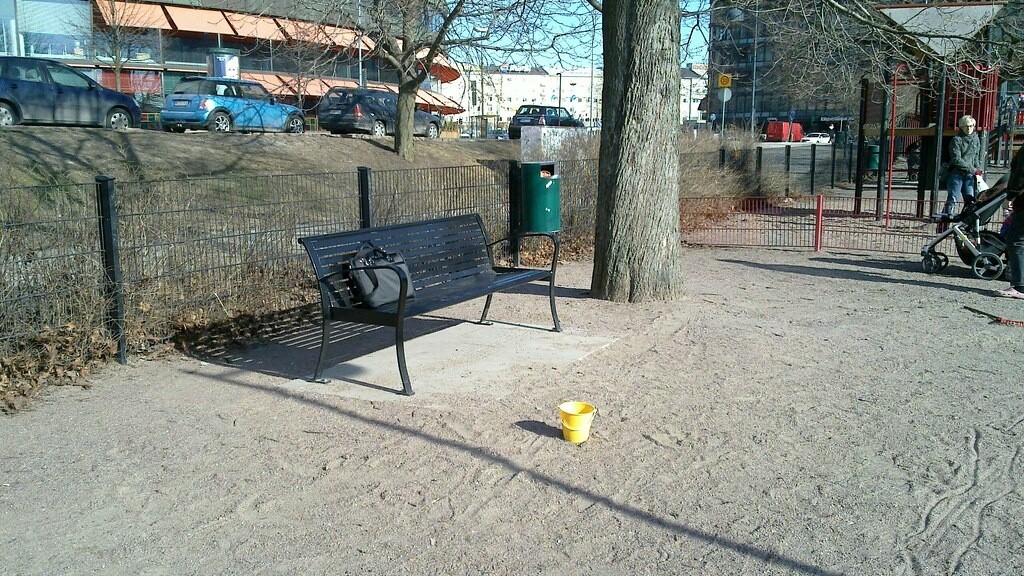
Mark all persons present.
[905,140,922,180]
[1018,93,1024,109]
[935,115,985,234]
[828,124,835,143]
[994,143,1024,300]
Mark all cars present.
[800,132,831,144]
[487,130,508,140]
[159,76,307,134]
[0,56,141,128]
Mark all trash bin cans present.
[866,145,880,170]
[509,158,561,239]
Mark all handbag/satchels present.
[348,243,416,308]
[976,175,989,193]
[940,162,951,182]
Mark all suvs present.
[508,104,585,140]
[317,86,443,138]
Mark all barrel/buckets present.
[559,402,596,445]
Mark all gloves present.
[975,170,983,176]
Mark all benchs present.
[298,212,563,397]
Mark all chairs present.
[10,68,21,80]
[224,88,233,95]
[25,68,41,82]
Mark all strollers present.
[920,172,1011,281]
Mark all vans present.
[766,121,806,142]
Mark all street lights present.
[473,26,488,120]
[556,73,561,126]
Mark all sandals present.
[995,287,1024,298]
